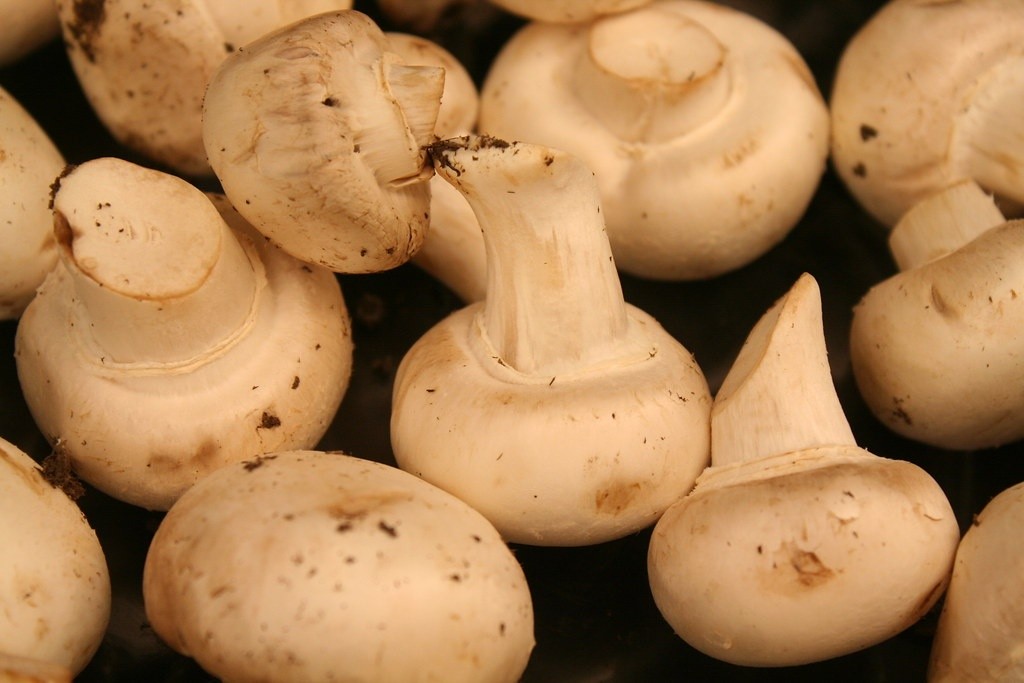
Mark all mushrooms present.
[0,0,1024,683]
